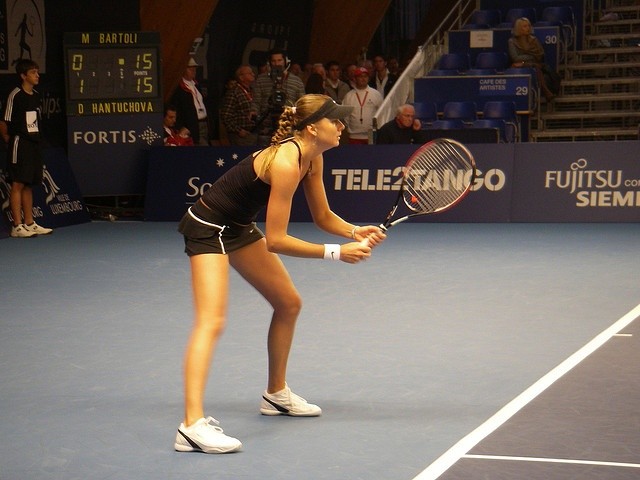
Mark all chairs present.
[461,4,574,46]
[410,101,522,143]
[429,51,544,95]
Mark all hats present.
[186,58,197,66]
[354,67,368,75]
[295,99,354,129]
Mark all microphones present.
[282,63,290,74]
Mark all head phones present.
[266,56,291,66]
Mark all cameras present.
[267,91,287,109]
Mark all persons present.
[341,67,384,125]
[246,51,307,148]
[175,56,212,147]
[367,56,398,98]
[162,106,178,138]
[322,60,351,103]
[177,95,389,453]
[387,58,402,74]
[258,57,273,72]
[312,61,327,80]
[220,66,271,149]
[345,63,359,89]
[373,104,422,145]
[388,57,402,75]
[406,55,412,63]
[291,63,307,79]
[1,59,54,238]
[219,78,237,146]
[304,62,317,80]
[163,126,195,146]
[507,17,553,98]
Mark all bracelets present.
[323,242,341,262]
[351,224,360,241]
[522,61,524,65]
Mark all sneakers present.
[23,221,53,235]
[260,385,322,416]
[174,418,242,454]
[11,224,37,238]
